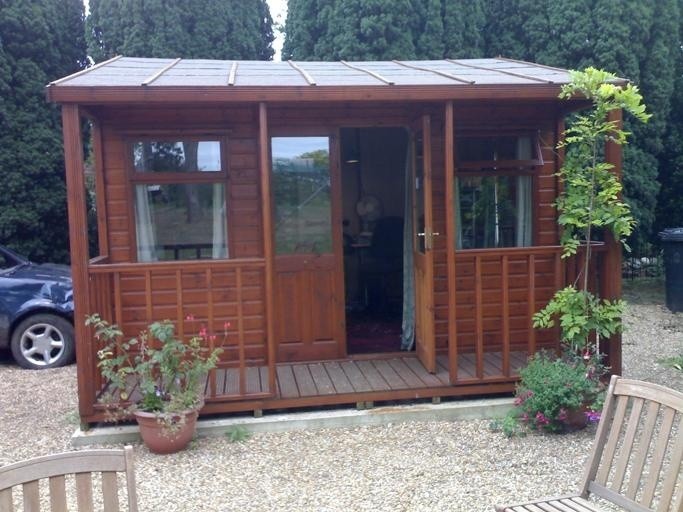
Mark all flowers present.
[513,342,613,433]
[85,313,231,440]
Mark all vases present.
[569,404,589,429]
[133,411,196,454]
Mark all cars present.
[621,257,660,279]
[0,243,75,369]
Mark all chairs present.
[0,445,138,512]
[495,374,683,512]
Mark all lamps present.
[345,129,363,199]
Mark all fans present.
[355,193,384,237]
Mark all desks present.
[350,243,371,307]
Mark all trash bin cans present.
[657,225,683,314]
